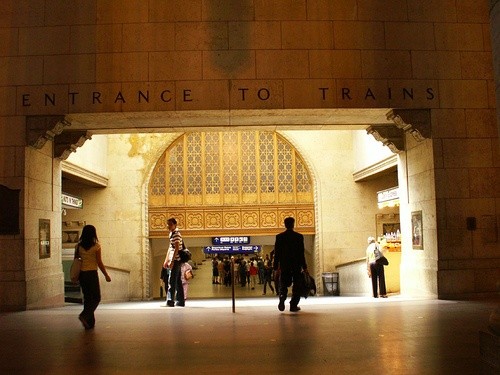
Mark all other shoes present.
[176,301,185,307]
[380,294,388,298]
[290,305,300,312]
[78,314,95,330]
[166,299,174,307]
[277,295,285,311]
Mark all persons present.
[159,249,279,301]
[273,217,308,312]
[160,217,186,307]
[74,225,111,330]
[366,236,388,298]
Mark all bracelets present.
[171,260,175,263]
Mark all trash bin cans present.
[322,272,341,296]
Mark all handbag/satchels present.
[185,269,192,280]
[300,271,316,298]
[376,255,388,265]
[69,242,82,282]
[178,248,192,262]
